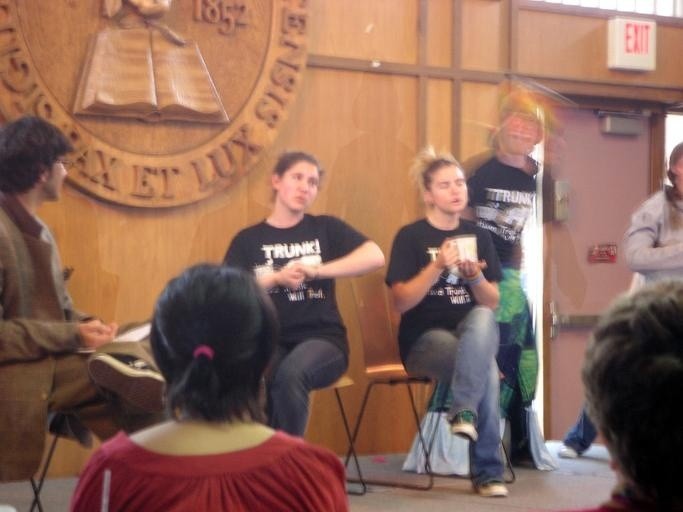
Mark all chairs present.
[311,373,367,496]
[342,268,515,491]
[27,395,114,512]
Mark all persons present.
[222,152,385,436]
[69,263,352,512]
[546,143,683,458]
[583,278,683,512]
[0,118,169,480]
[383,151,509,495]
[461,100,542,499]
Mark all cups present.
[455,234,478,265]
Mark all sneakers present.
[451,412,478,443]
[87,353,165,412]
[558,444,578,459]
[477,483,507,497]
